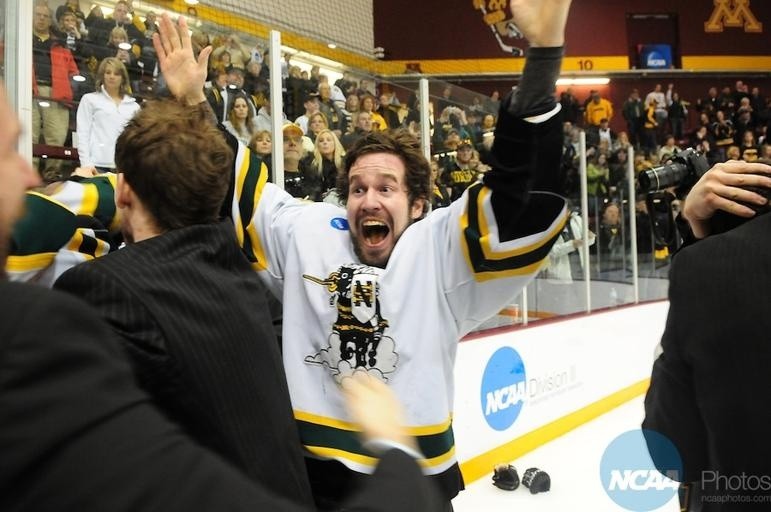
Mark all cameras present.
[639,148,710,201]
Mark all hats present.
[283,124,304,135]
[454,138,471,149]
[303,89,320,100]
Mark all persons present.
[642,211,769,512]
[153,0,575,511]
[52,94,318,511]
[2,72,456,511]
[1,1,771,258]
